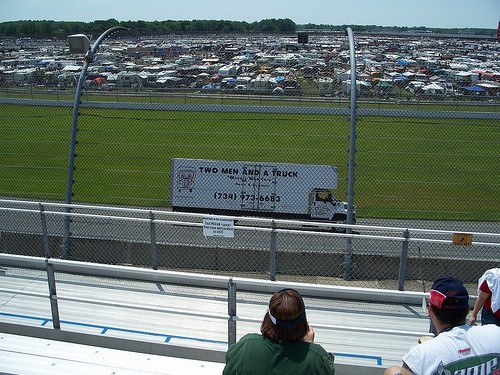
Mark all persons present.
[384,268,500,375]
[470,268,500,327]
[222,288,336,375]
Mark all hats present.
[268,288,306,327]
[430,275,470,310]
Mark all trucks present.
[169,156,357,233]
